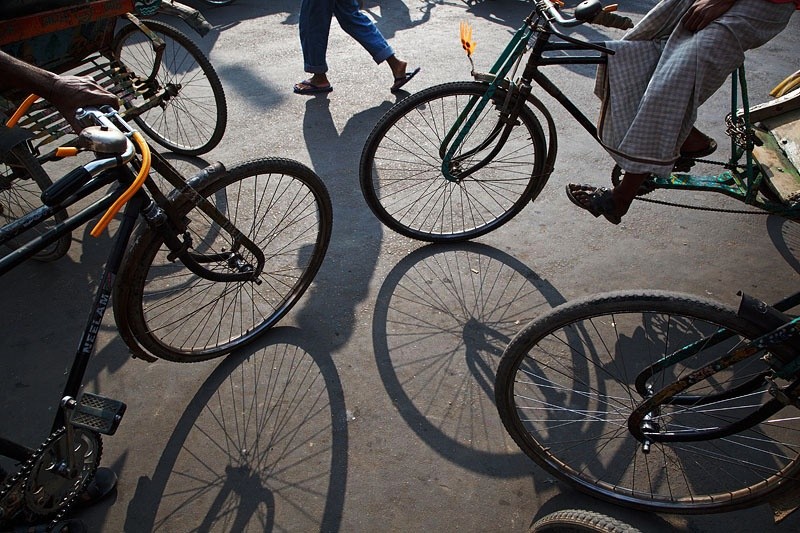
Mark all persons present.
[566,0,800,225]
[293,0,421,93]
[0,51,119,524]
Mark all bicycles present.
[0,102,334,533]
[492,291,799,516]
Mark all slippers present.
[681,136,718,157]
[566,183,621,224]
[391,67,421,91]
[294,80,333,93]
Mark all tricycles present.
[0,0,228,263]
[358,0,800,246]
